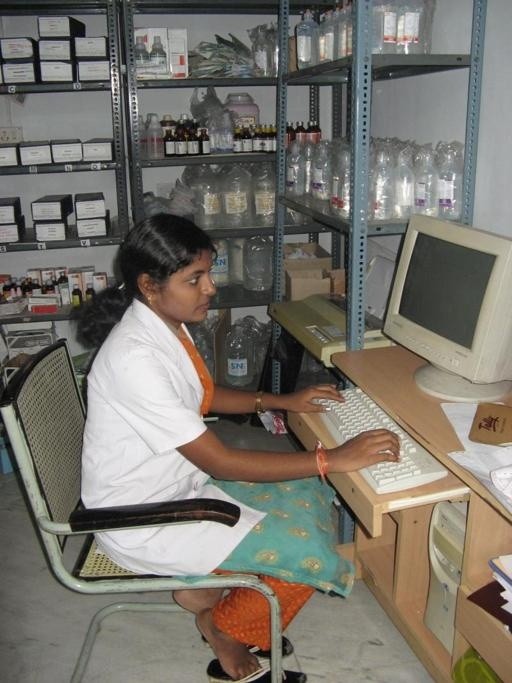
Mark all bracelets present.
[315,440,327,481]
[255,390,264,414]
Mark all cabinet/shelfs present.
[1,0,129,386]
[120,1,281,311]
[272,0,487,543]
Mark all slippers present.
[205,657,307,682]
[201,635,293,659]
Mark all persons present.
[71,212,401,683]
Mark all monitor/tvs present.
[382,212,511,402]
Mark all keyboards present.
[310,386,449,493]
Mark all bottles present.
[222,92,260,131]
[209,237,228,287]
[228,235,243,285]
[161,115,177,131]
[165,130,175,156]
[176,130,187,155]
[179,114,188,125]
[254,26,267,76]
[225,325,252,386]
[264,235,274,247]
[200,129,211,154]
[221,164,253,227]
[294,3,426,70]
[132,36,150,75]
[267,27,278,77]
[254,170,274,226]
[187,130,199,155]
[243,234,274,292]
[285,121,466,222]
[2,271,96,309]
[149,36,167,74]
[234,123,277,153]
[190,164,221,231]
[146,115,165,160]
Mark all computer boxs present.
[421,501,467,654]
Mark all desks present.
[286,343,512,683]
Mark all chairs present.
[1,338,281,683]
